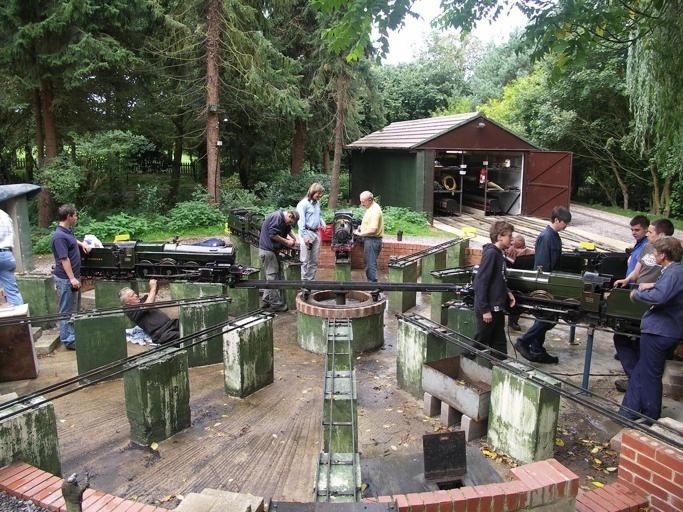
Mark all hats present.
[290,209,300,224]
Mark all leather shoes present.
[514,340,537,362]
[509,320,521,330]
[531,348,559,365]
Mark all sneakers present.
[615,378,629,391]
[261,304,287,312]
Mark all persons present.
[119,278,181,348]
[459,222,513,368]
[512,206,573,363]
[352,190,384,282]
[611,214,683,428]
[258,207,299,312]
[51,204,92,351]
[295,182,327,291]
[0,207,23,307]
[504,234,530,331]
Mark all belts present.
[0,249,12,252]
[306,227,319,232]
